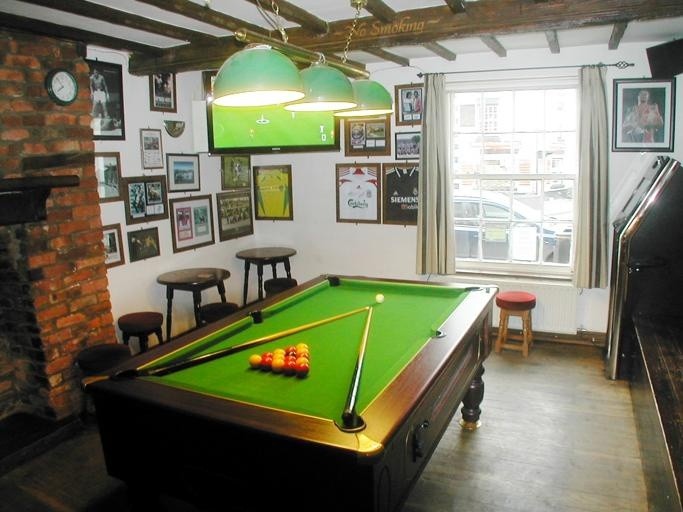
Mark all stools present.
[495,291,536,357]
[75,310,164,373]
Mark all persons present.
[410,89,420,117]
[177,211,189,226]
[131,182,163,216]
[402,91,414,118]
[154,74,163,96]
[130,234,143,253]
[218,156,250,225]
[623,88,664,144]
[144,231,158,253]
[88,68,121,133]
[160,72,171,98]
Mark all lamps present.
[212,0,393,119]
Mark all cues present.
[144,305,370,376]
[342,307,373,419]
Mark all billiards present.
[247,342,310,377]
[374,293,387,305]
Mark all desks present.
[157,247,297,342]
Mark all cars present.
[452,188,576,265]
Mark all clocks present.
[45,69,78,106]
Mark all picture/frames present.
[612,77,676,152]
[336,83,424,227]
